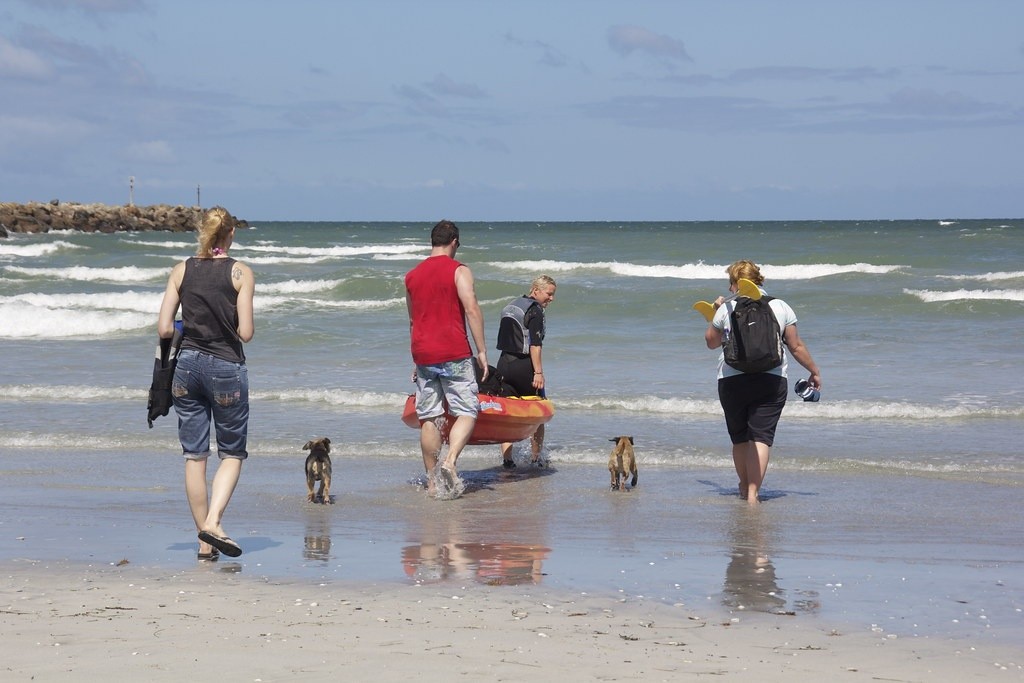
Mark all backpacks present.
[721,296,784,374]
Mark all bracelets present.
[534,372,543,374]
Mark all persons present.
[156,206,255,560]
[405,219,489,501]
[497,275,556,469]
[704,260,821,504]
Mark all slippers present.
[803,389,820,403]
[199,530,243,557]
[795,378,814,401]
[196,548,221,563]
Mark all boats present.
[405,391,553,446]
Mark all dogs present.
[608,436,638,492]
[302,437,332,505]
